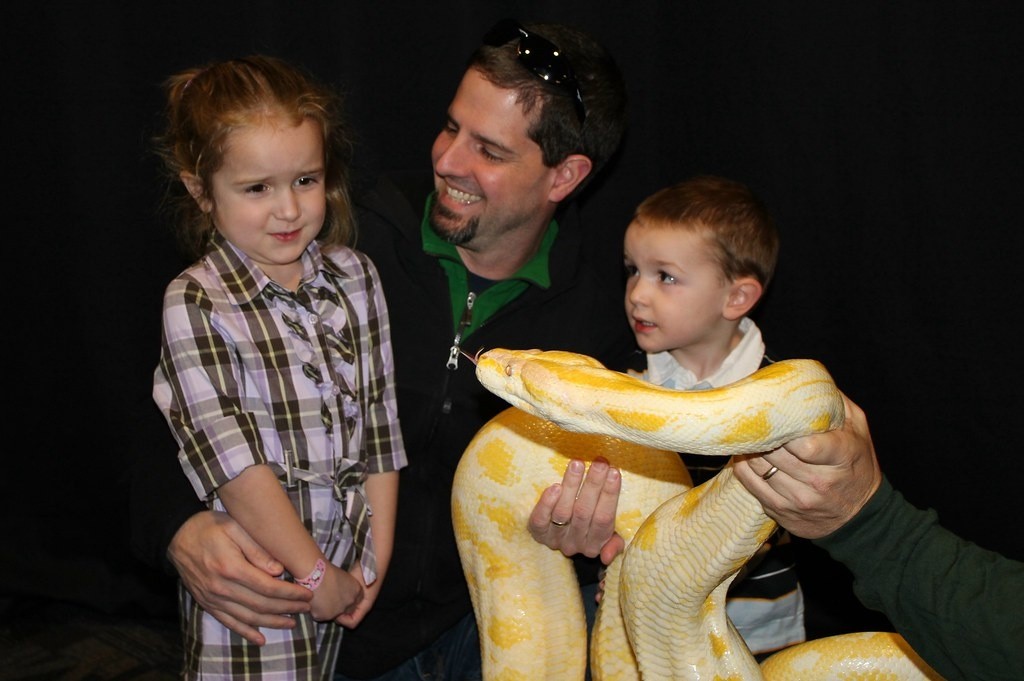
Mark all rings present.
[550,519,571,528]
[763,466,779,482]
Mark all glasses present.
[482,17,591,157]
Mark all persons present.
[623,175,809,661]
[150,23,644,681]
[733,390,1024,680]
[151,57,410,681]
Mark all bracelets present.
[293,559,327,591]
